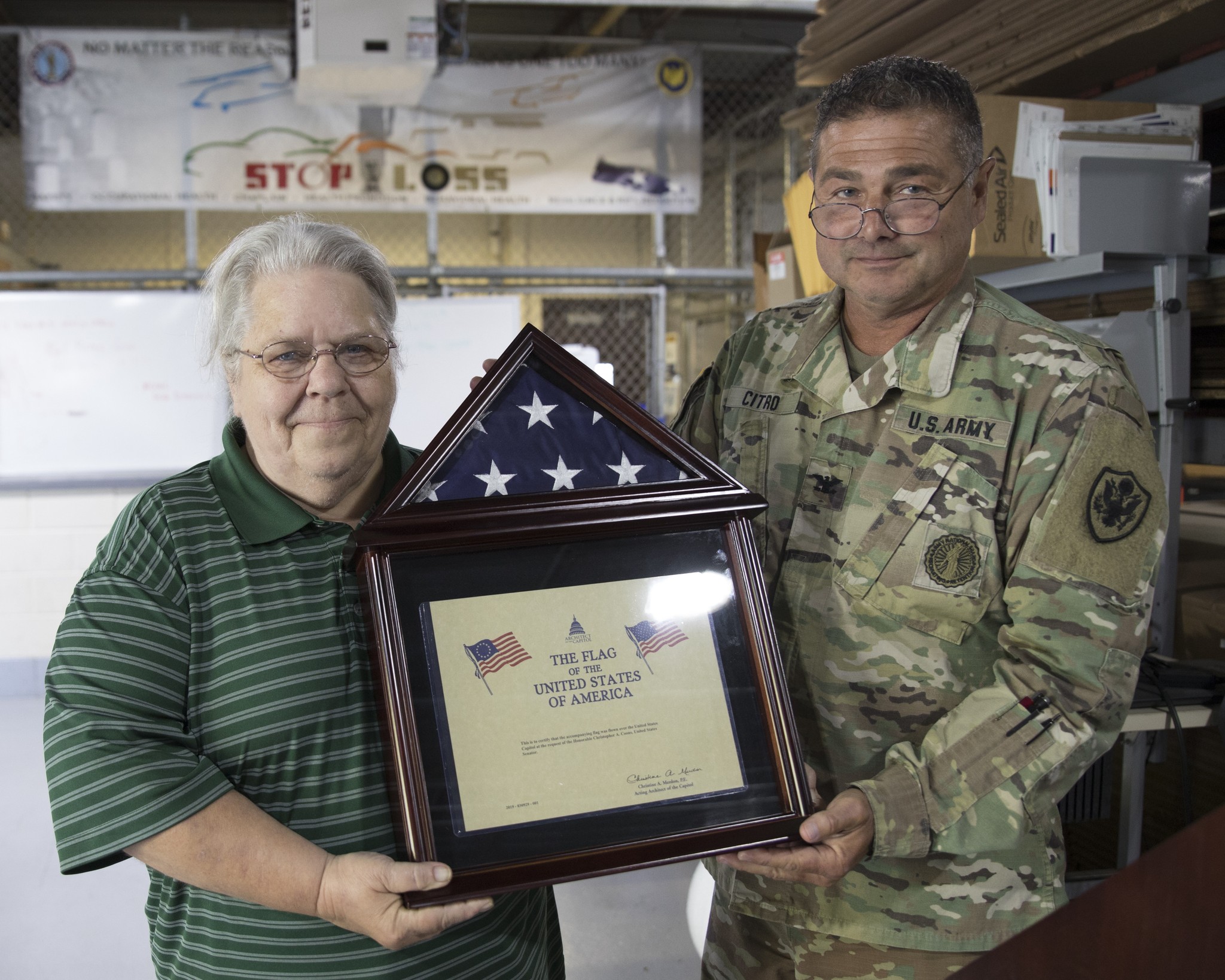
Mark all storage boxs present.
[764,87,1203,309]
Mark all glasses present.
[235,334,397,380]
[808,164,980,240]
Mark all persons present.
[465,58,1167,980]
[40,221,564,980]
